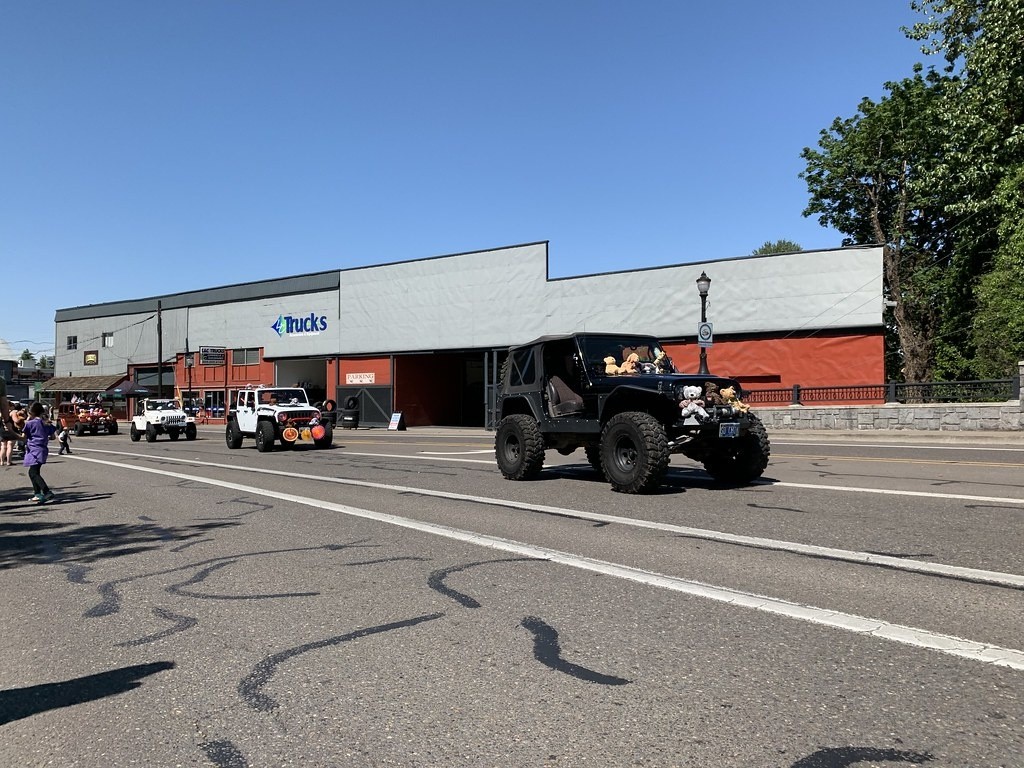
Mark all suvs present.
[132,397,197,443]
[56,401,118,435]
[495,332,770,495]
[226,385,335,454]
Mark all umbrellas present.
[100,380,161,421]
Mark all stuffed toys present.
[79,405,113,423]
[622,352,640,372]
[604,357,621,374]
[679,385,710,417]
[290,398,298,405]
[705,382,726,405]
[720,386,749,413]
[654,351,667,374]
[269,398,278,406]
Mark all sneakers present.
[36,490,54,505]
[28,493,43,501]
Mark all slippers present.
[7,462,17,466]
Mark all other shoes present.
[58,452,64,455]
[67,451,72,453]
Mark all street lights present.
[697,270,712,375]
[186,355,195,418]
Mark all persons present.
[0,377,39,465]
[58,428,71,456]
[6,402,56,504]
